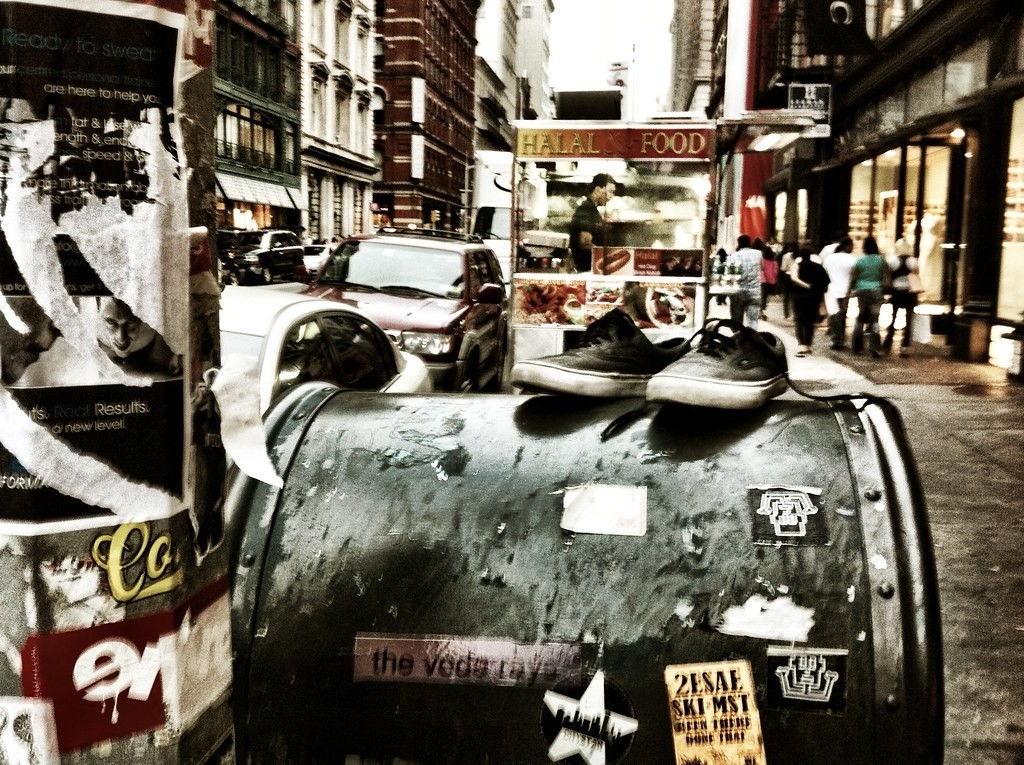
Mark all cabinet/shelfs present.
[1004,166,1024,241]
[850,204,917,242]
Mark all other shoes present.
[795,345,810,356]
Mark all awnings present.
[715,109,815,154]
[215,169,305,209]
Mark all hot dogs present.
[594,249,630,274]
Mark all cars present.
[305,243,347,279]
[220,228,305,284]
[219,281,433,531]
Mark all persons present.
[905,213,943,298]
[714,234,919,358]
[81,295,156,359]
[568,173,617,272]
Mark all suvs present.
[301,229,507,393]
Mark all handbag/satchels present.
[908,274,924,294]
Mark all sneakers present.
[509,307,689,399]
[646,318,788,412]
